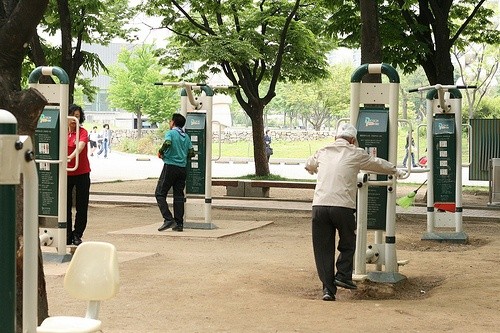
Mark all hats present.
[339,123,358,147]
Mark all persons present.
[304,123,404,301]
[88,124,112,158]
[402,129,418,168]
[67,104,92,245]
[155,112,196,232]
[264,129,271,165]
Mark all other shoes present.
[72,231,81,245]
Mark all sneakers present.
[172,224,183,231]
[322,287,335,300]
[333,273,357,289]
[157,220,177,231]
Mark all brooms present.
[396,179,428,210]
